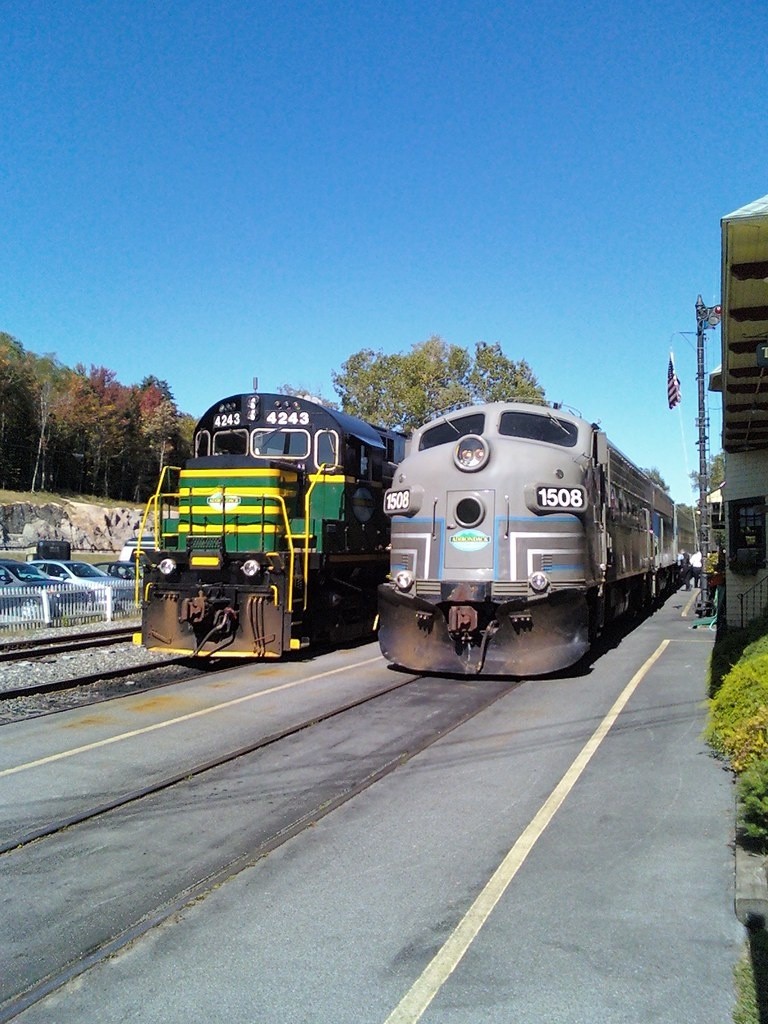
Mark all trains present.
[124,391,409,665]
[367,398,702,681]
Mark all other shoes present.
[689,585,692,591]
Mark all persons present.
[689,550,702,587]
[680,549,693,592]
[0,523,11,551]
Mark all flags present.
[667,358,682,409]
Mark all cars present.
[0,558,96,620]
[120,536,156,574]
[17,559,128,614]
[78,560,145,601]
